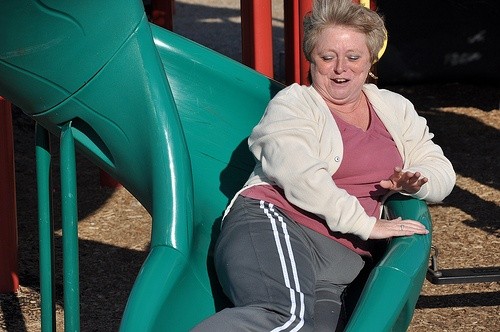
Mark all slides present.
[0,0,433,332]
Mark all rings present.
[401,225,404,231]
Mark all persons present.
[190,0,457,332]
